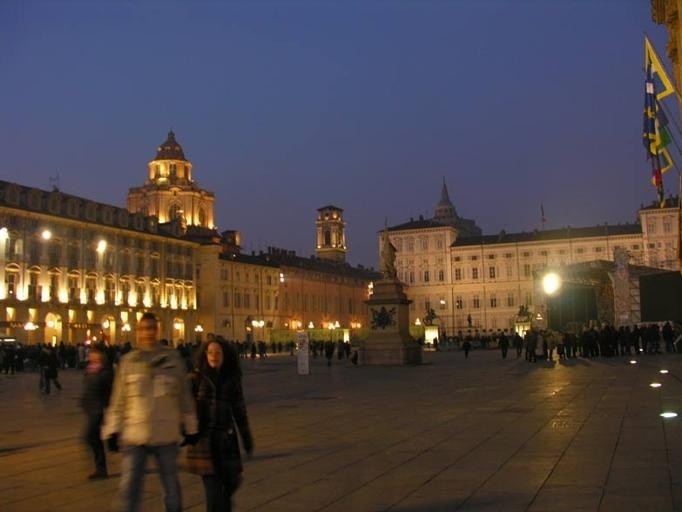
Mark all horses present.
[423,308,441,326]
[512,305,533,323]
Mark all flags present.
[640,37,674,209]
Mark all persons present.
[434,337,440,352]
[161,332,231,373]
[187,335,252,512]
[496,322,680,362]
[101,313,200,511]
[308,339,358,366]
[231,340,295,359]
[80,346,119,480]
[2,342,132,392]
[461,339,471,358]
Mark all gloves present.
[107,434,118,454]
[180,434,201,448]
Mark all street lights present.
[252,321,264,340]
[193,325,204,342]
[24,322,37,344]
[120,323,132,344]
[328,322,335,343]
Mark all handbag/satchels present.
[176,441,221,476]
